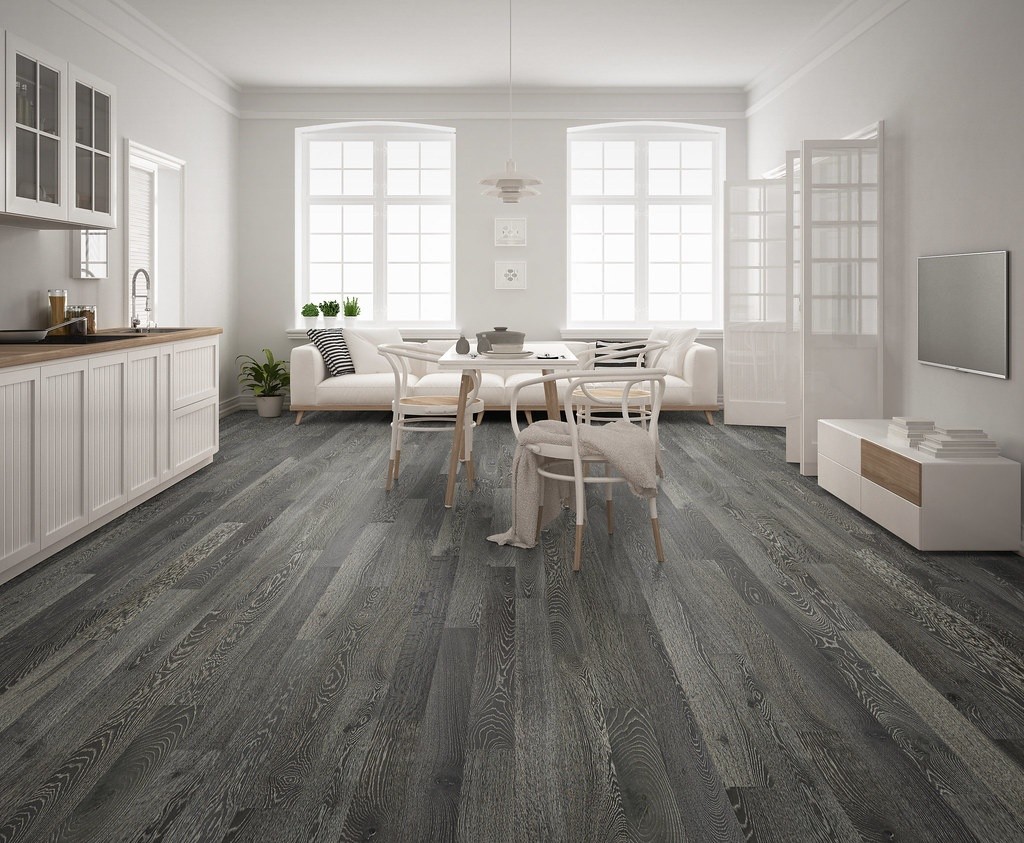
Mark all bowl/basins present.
[491,343,523,353]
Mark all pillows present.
[594,340,650,369]
[343,327,411,374]
[307,328,355,377]
[645,328,700,377]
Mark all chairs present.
[376,344,485,509]
[567,341,670,440]
[510,367,670,572]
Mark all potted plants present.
[301,303,320,329]
[235,348,290,417]
[318,300,340,329]
[342,296,360,328]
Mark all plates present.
[481,350,534,358]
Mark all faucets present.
[129,269,152,329]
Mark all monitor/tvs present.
[917,250,1010,380]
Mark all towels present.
[485,419,658,549]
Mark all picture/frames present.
[494,262,527,290]
[494,217,526,247]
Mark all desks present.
[437,343,595,509]
[290,340,721,426]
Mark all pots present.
[0,315,86,342]
[476,327,525,356]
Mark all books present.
[887,415,1003,459]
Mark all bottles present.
[48,289,66,335]
[80,305,97,334]
[456,336,470,354]
[66,305,80,334]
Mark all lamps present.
[479,0,542,204]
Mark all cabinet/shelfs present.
[817,419,1021,551]
[0,335,220,587]
[0,29,118,231]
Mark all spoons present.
[470,353,478,358]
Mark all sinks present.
[122,327,196,333]
